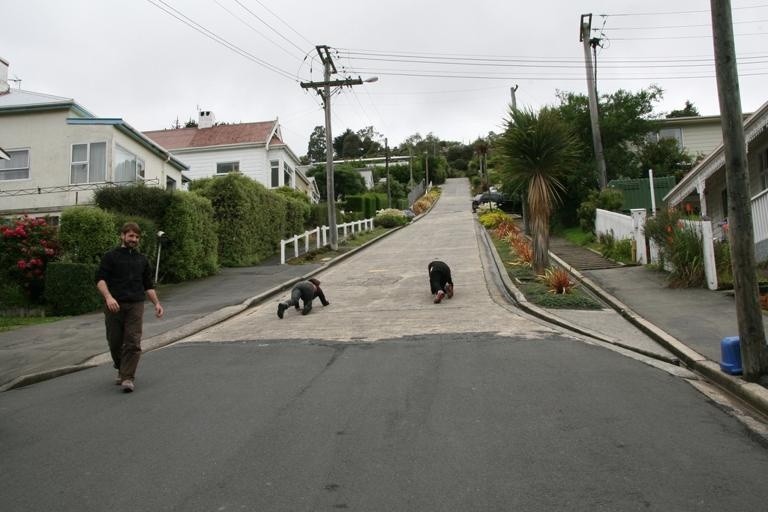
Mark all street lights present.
[323,76,381,253]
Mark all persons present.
[429,257,453,303]
[277,278,329,319]
[95,222,164,391]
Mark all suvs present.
[472,192,524,214]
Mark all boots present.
[277,303,287,319]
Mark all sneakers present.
[446,284,453,299]
[115,369,121,385]
[434,290,445,304]
[120,380,134,392]
